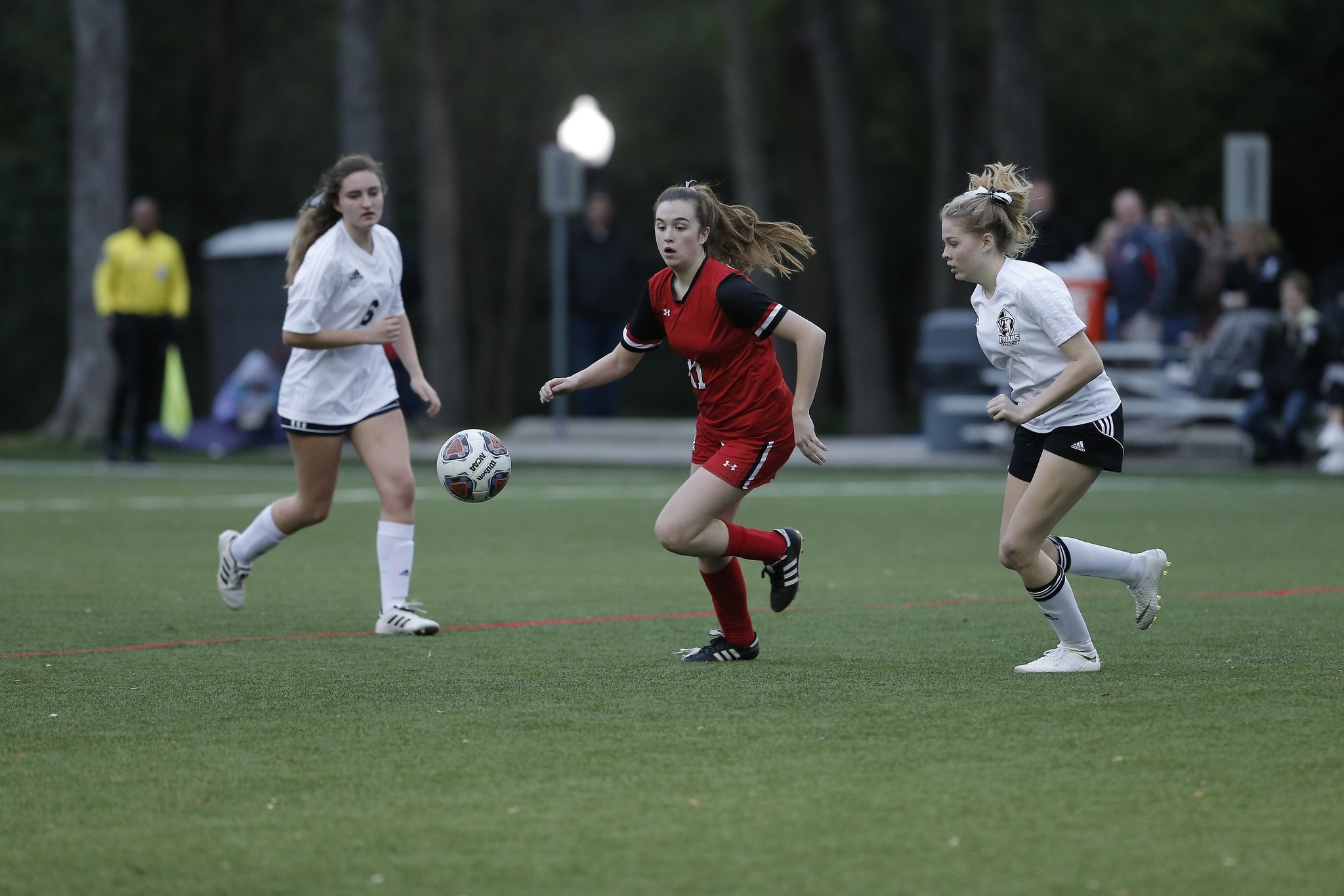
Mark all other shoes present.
[1279,439,1306,463]
[1321,451,1344,475]
[1254,436,1280,463]
[1318,424,1344,448]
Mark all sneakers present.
[1015,649,1102,673]
[683,629,760,662]
[216,529,255,610]
[761,527,804,612]
[1127,548,1170,630]
[376,602,439,636]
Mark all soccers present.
[436,427,512,504]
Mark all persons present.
[94,195,190,463]
[1019,171,1076,271]
[1085,187,1344,479]
[540,181,829,662]
[939,161,1172,672]
[219,154,439,636]
